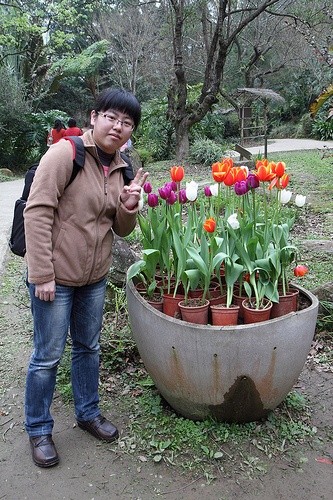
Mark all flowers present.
[126,158,306,310]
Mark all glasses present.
[97,112,135,132]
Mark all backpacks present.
[8,135,132,257]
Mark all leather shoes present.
[29,434,58,468]
[77,415,118,442]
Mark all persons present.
[23,90,149,467]
[48,119,131,152]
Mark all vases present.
[135,262,299,326]
[126,261,319,423]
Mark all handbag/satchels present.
[46,129,53,147]
